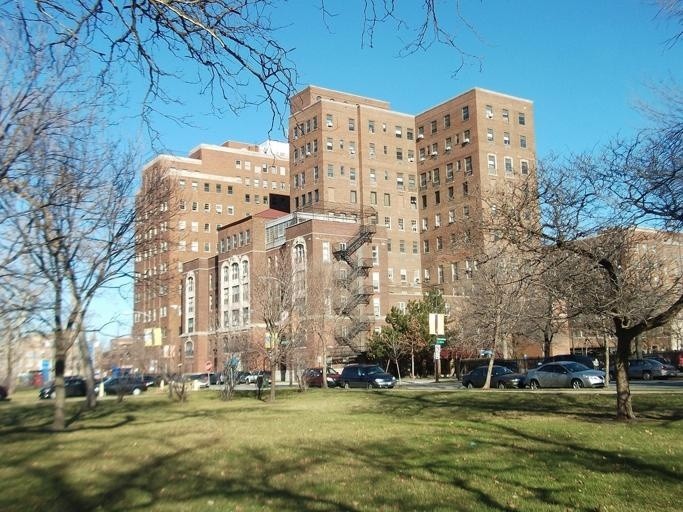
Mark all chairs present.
[313,369,320,376]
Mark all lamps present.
[418,157,426,162]
[461,138,469,144]
[444,147,451,151]
[417,133,423,138]
[431,151,437,155]
[397,178,403,182]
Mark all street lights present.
[415,281,440,384]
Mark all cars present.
[38,377,86,400]
[50,373,155,396]
[608,354,683,381]
[300,368,321,386]
[475,347,493,358]
[0,383,8,400]
[460,364,525,389]
[156,370,250,388]
[303,367,340,388]
[337,363,397,389]
[523,360,610,390]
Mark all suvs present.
[243,369,271,385]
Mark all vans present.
[534,353,603,370]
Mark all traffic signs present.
[436,340,444,344]
